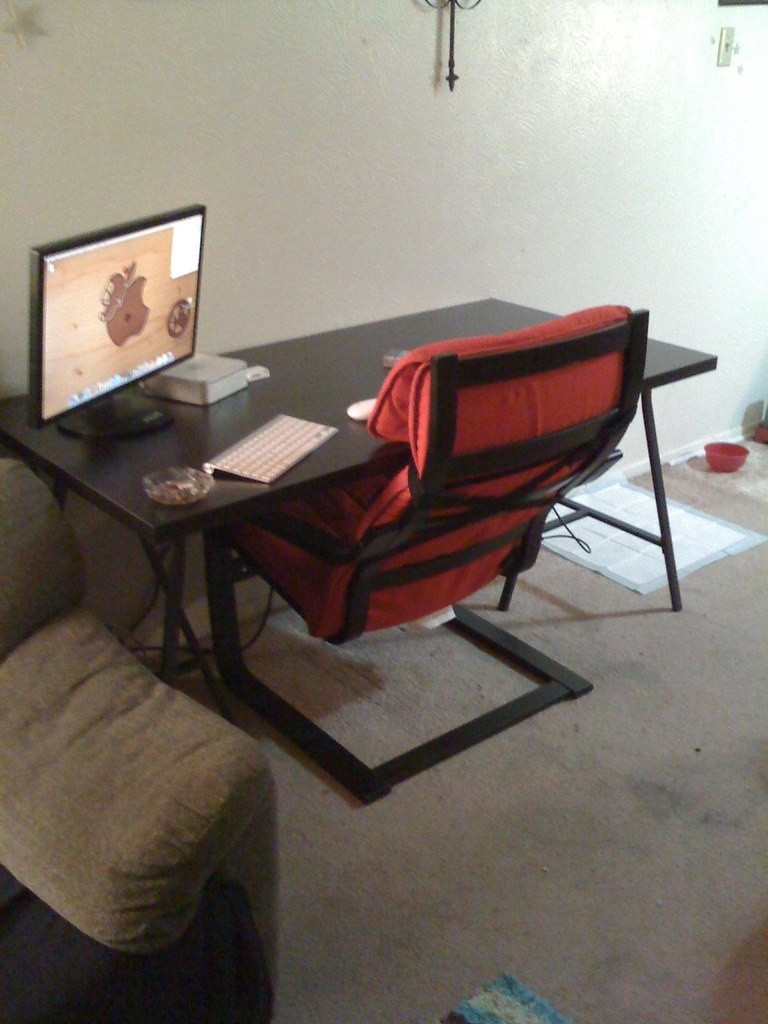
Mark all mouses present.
[348,398,378,420]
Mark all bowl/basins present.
[704,441,750,472]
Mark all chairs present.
[203,304,649,804]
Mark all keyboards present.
[204,415,339,484]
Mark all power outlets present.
[717,26,734,67]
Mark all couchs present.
[0,459,281,1024]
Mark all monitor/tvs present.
[28,203,204,441]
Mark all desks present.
[0,297,718,727]
